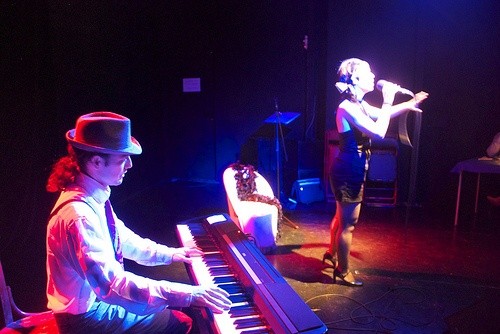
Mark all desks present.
[449,160,500,227]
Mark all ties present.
[106,200,124,264]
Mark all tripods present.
[275,97,299,228]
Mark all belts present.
[53,313,72,318]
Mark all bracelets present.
[382,102,391,105]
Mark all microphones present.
[376,79,414,97]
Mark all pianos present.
[175,212,328,334]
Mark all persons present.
[325,59,428,285]
[46,112,230,333]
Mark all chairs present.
[0,262,61,334]
[223,167,278,254]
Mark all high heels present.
[333,268,363,286]
[323,252,336,266]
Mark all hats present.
[65,112,142,155]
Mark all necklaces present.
[356,100,368,115]
